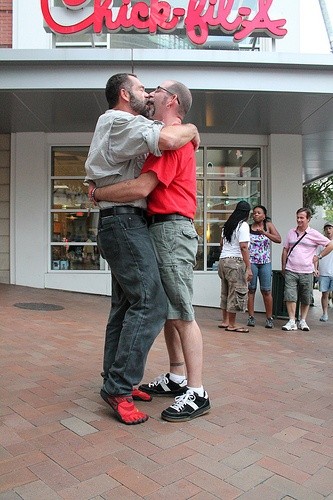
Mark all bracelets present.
[91,187,97,197]
[247,266,251,269]
[317,253,322,259]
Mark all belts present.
[148,213,191,224]
[99,206,146,217]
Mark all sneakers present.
[247,317,255,327]
[298,319,309,331]
[265,317,274,328]
[282,319,297,330]
[132,388,153,401]
[100,390,147,425]
[137,373,187,398]
[320,314,329,321]
[161,390,211,422]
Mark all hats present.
[324,222,333,227]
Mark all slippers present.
[217,326,228,328]
[224,327,249,332]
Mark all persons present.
[84,80,211,422]
[77,72,201,425]
[218,200,254,333]
[281,208,333,331]
[314,221,333,322]
[247,205,282,330]
